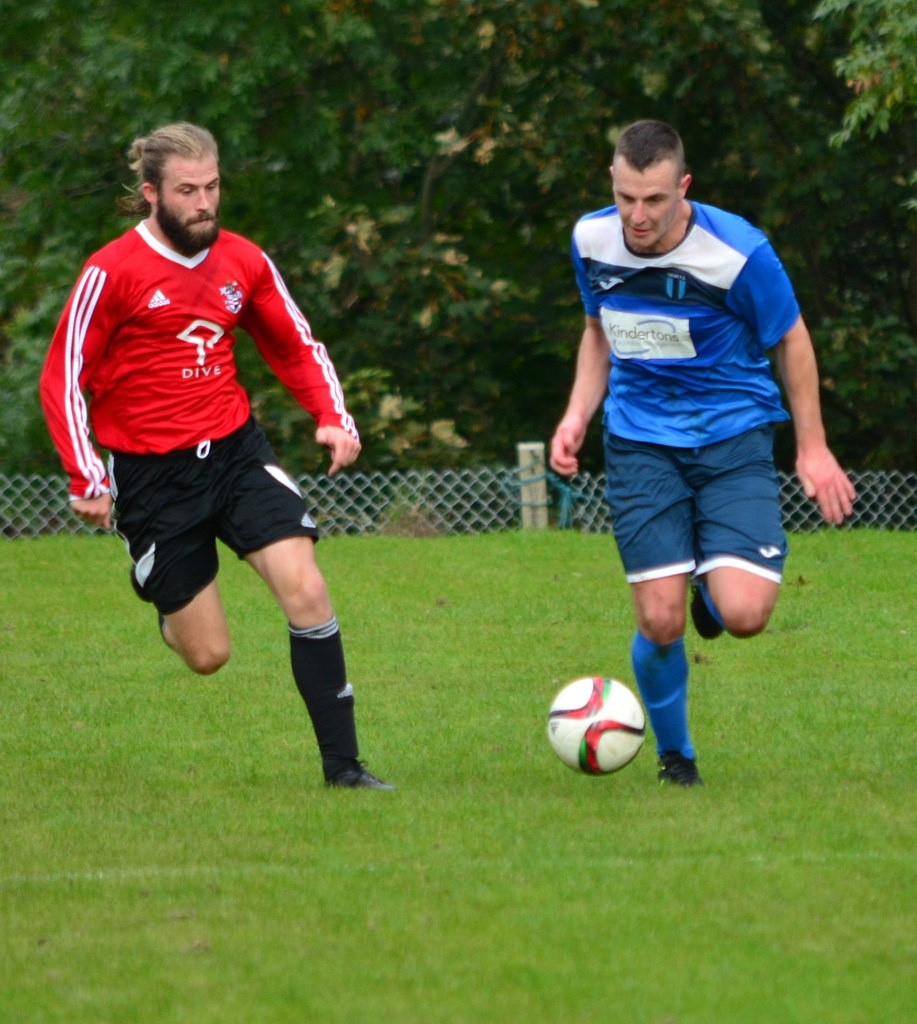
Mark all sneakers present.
[657,755,703,787]
[690,580,724,640]
[325,767,393,790]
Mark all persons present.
[38,121,390,788]
[549,120,859,788]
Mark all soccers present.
[546,675,648,777]
